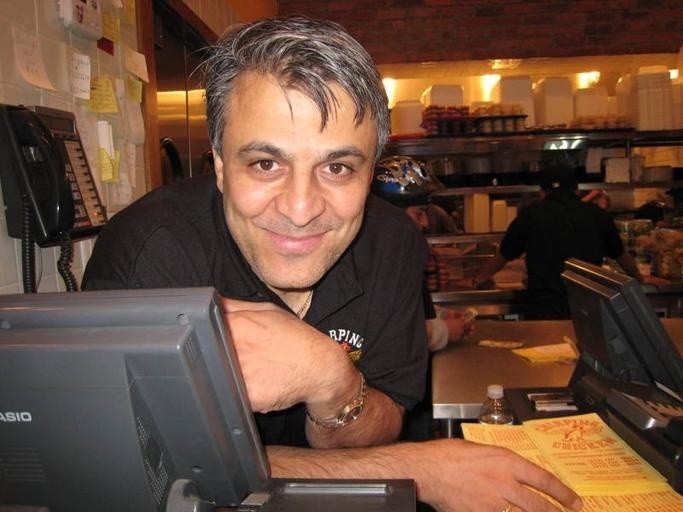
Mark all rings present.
[502,503,514,512]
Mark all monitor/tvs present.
[558,256,683,399]
[0,287,272,512]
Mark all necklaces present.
[290,289,312,319]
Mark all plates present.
[628,71,675,131]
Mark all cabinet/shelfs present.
[384,128,683,317]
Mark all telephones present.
[0,104,108,248]
[160,137,184,186]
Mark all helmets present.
[369,153,447,204]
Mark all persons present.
[80,11,585,512]
[578,188,609,209]
[370,153,475,353]
[453,146,642,318]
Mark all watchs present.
[301,371,366,432]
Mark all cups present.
[569,112,626,130]
[421,103,526,137]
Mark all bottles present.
[478,384,514,428]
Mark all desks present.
[423,317,680,440]
